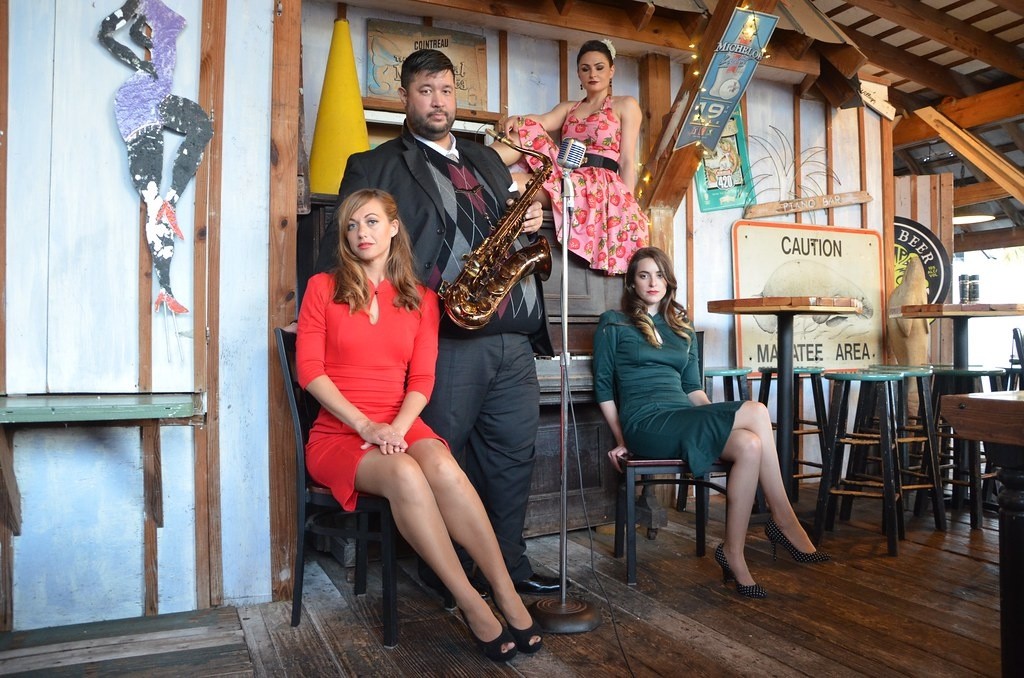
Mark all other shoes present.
[458,599,517,662]
[489,585,542,653]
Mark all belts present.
[580,153,618,172]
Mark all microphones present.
[555,137,586,219]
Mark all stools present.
[812,371,905,559]
[677,366,767,512]
[757,366,827,512]
[840,359,1024,532]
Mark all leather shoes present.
[435,575,487,598]
[514,573,571,596]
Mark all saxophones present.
[436,128,555,332]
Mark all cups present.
[959,275,980,305]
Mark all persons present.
[309,50,574,608]
[593,247,833,600]
[294,189,543,663]
[489,37,650,277]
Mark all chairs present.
[614,332,708,585]
[273,326,457,648]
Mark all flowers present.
[600,38,617,60]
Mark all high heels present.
[763,518,831,563]
[715,542,768,600]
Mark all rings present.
[391,432,395,436]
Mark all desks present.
[707,296,863,536]
[942,390,1024,678]
[889,303,1024,512]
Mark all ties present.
[446,151,459,163]
